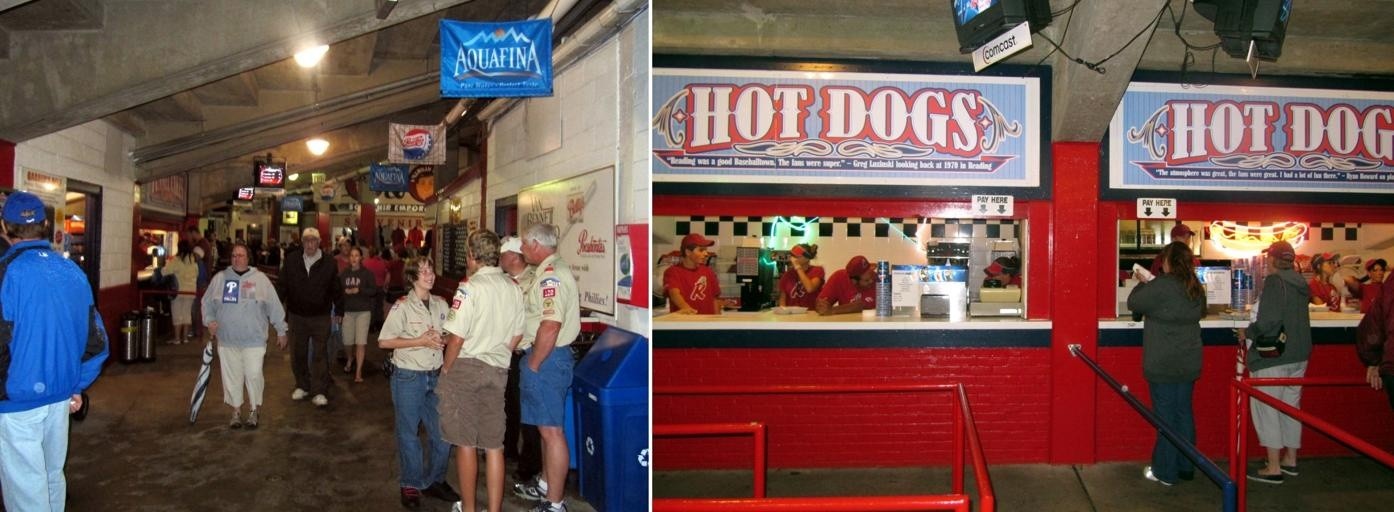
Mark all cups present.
[1233,270,1253,311]
[875,261,892,316]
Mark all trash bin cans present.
[120,310,158,365]
[572,327,648,512]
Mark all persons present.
[0,191,582,512]
[779,243,824,310]
[982,257,1022,286]
[1151,225,1200,275]
[815,255,878,315]
[1309,252,1394,412]
[663,233,731,316]
[1238,242,1313,483]
[1127,242,1207,486]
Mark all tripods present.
[232,186,255,201]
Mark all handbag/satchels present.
[162,274,178,300]
[386,286,407,304]
[1255,325,1287,358]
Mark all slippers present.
[1264,460,1299,476]
[1246,469,1284,484]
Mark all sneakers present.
[1144,465,1176,486]
[483,448,568,512]
[336,350,364,383]
[291,386,328,406]
[230,409,258,428]
[168,331,194,344]
[402,481,462,512]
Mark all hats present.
[984,260,1016,276]
[301,227,320,243]
[1312,252,1340,265]
[4,189,47,224]
[681,234,714,247]
[500,237,523,254]
[1171,224,1195,238]
[1364,259,1386,270]
[847,256,877,276]
[1262,241,1295,261]
[193,246,205,259]
[791,246,812,258]
[337,236,351,245]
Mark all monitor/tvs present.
[254,161,285,188]
[1192,0,1292,62]
[949,0,1053,54]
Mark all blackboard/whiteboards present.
[517,165,615,316]
